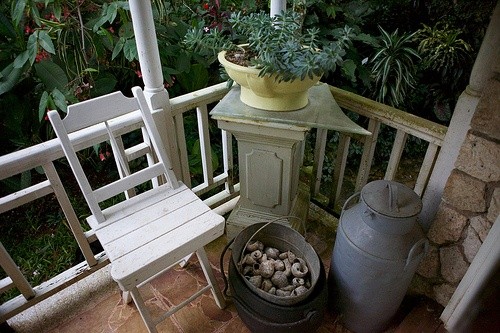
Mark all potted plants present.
[181,9,356,112]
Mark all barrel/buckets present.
[328,179,425,333]
[229,221,327,332]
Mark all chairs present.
[47,86,225,333]
